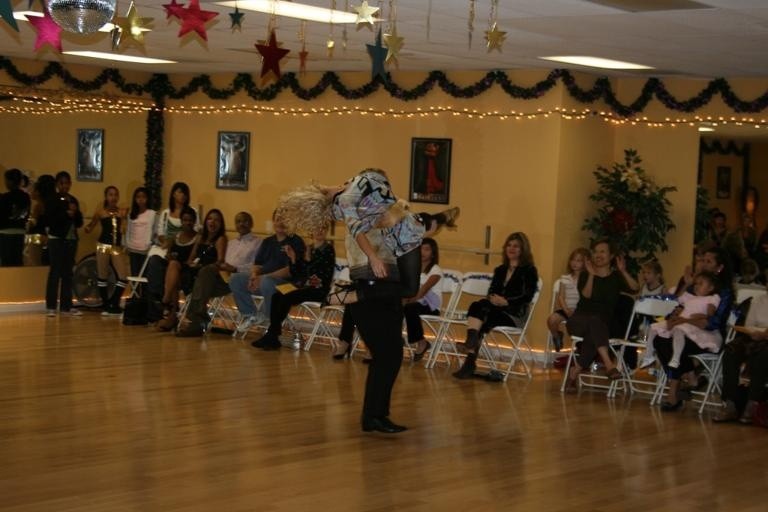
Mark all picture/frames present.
[409,137,451,204]
[716,166,731,200]
[215,130,250,191]
[76,129,103,182]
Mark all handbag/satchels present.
[121,282,149,325]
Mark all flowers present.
[580,148,677,255]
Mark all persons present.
[44,171,84,316]
[0,168,56,267]
[452,231,538,380]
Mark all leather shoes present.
[362,415,408,433]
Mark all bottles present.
[638,315,651,344]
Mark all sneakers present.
[176,308,212,338]
[712,399,737,424]
[679,375,707,394]
[318,281,355,310]
[237,313,270,332]
[660,399,684,413]
[562,367,579,394]
[412,339,432,361]
[604,365,624,380]
[43,306,123,319]
[738,397,758,425]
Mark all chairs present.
[120,245,543,385]
[545,277,750,415]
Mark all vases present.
[613,211,636,230]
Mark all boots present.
[454,327,480,356]
[451,352,477,380]
[251,325,281,351]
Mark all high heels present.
[157,299,180,334]
[441,205,461,229]
[362,355,372,365]
[331,341,352,361]
